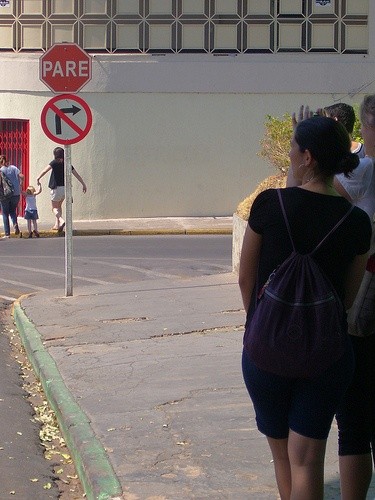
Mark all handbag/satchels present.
[0,170,15,200]
[48,160,61,190]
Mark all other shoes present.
[1,221,65,238]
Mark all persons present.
[287,94,375,499]
[37,147,87,232]
[323,103,366,160]
[20,184,42,238]
[0,155,24,239]
[238,116,373,500]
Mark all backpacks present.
[242,188,360,378]
